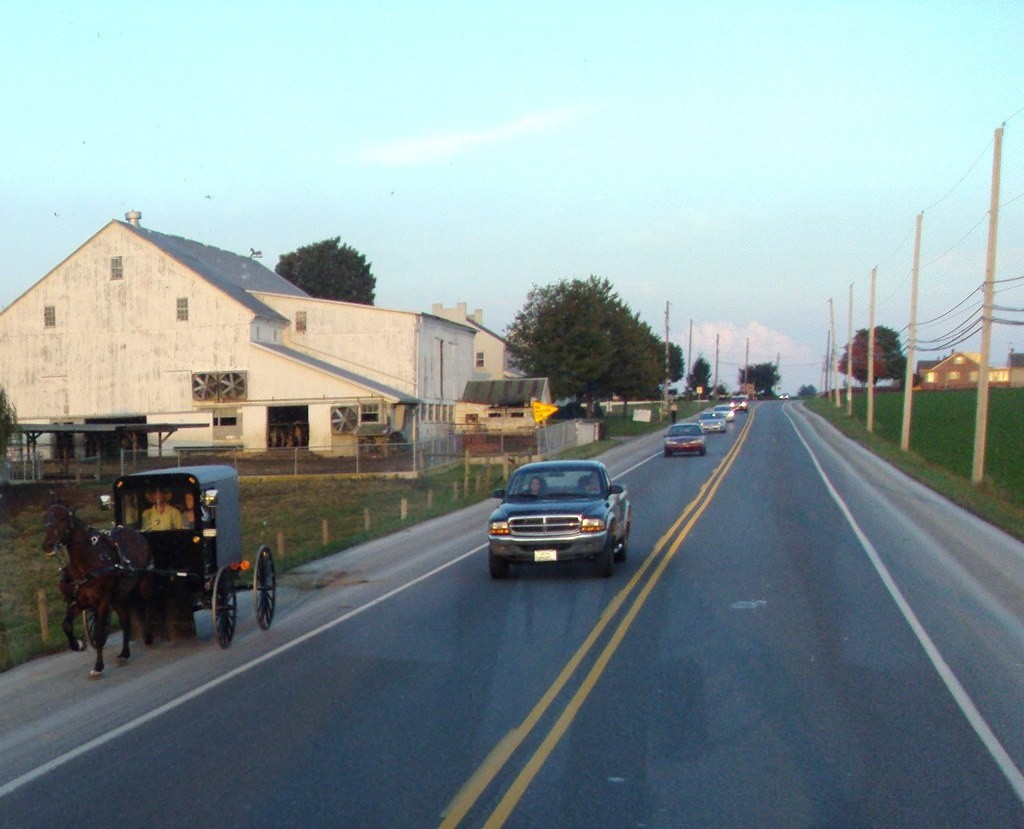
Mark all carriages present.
[40,465,278,677]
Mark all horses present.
[40,500,162,679]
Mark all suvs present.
[488,459,631,579]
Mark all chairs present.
[540,475,589,492]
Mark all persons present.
[182,493,194,529]
[529,477,541,495]
[669,400,677,424]
[587,473,600,492]
[139,489,183,532]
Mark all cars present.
[730,395,748,413]
[712,404,735,422]
[694,410,728,433]
[663,422,709,457]
